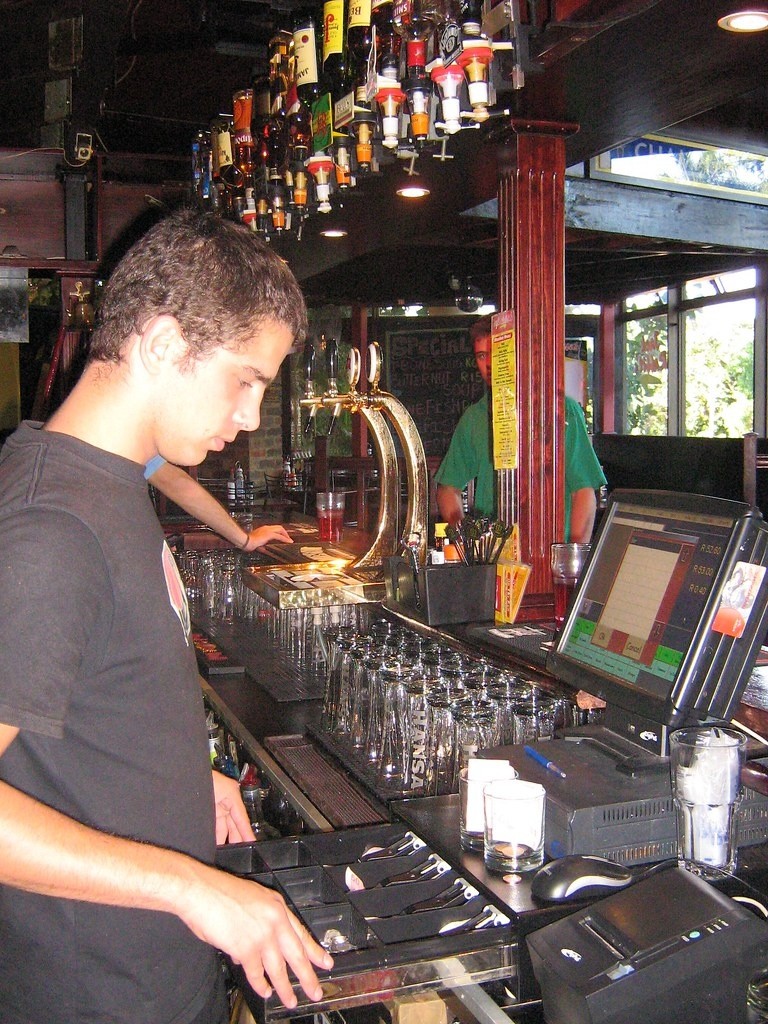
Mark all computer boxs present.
[476,740,768,868]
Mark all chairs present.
[198,473,309,514]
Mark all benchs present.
[588,432,768,523]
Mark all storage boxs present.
[382,556,497,627]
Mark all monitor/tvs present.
[545,487,768,778]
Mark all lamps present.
[456,277,485,314]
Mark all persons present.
[434,309,600,549]
[0,207,334,1024]
[143,455,294,552]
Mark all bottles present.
[190,1,493,229]
[283,462,291,487]
[227,466,236,507]
[172,547,377,677]
[237,464,245,499]
[205,720,314,840]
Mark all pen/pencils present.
[523,743,567,778]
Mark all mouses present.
[531,854,633,904]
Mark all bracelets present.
[242,532,250,549]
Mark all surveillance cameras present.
[74,146,90,161]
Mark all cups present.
[669,726,749,882]
[458,767,520,851]
[483,788,546,875]
[319,618,602,799]
[316,492,346,544]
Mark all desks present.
[224,497,296,512]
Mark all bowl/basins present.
[550,543,592,643]
